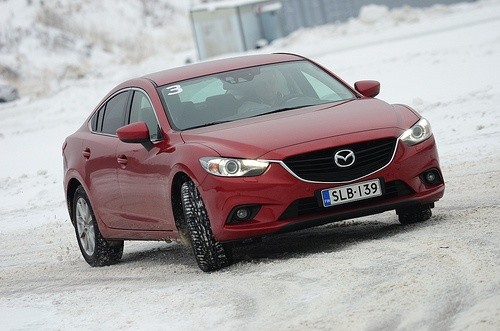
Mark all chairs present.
[140,94,236,138]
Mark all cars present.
[61,51,446,274]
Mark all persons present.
[236,75,298,116]
